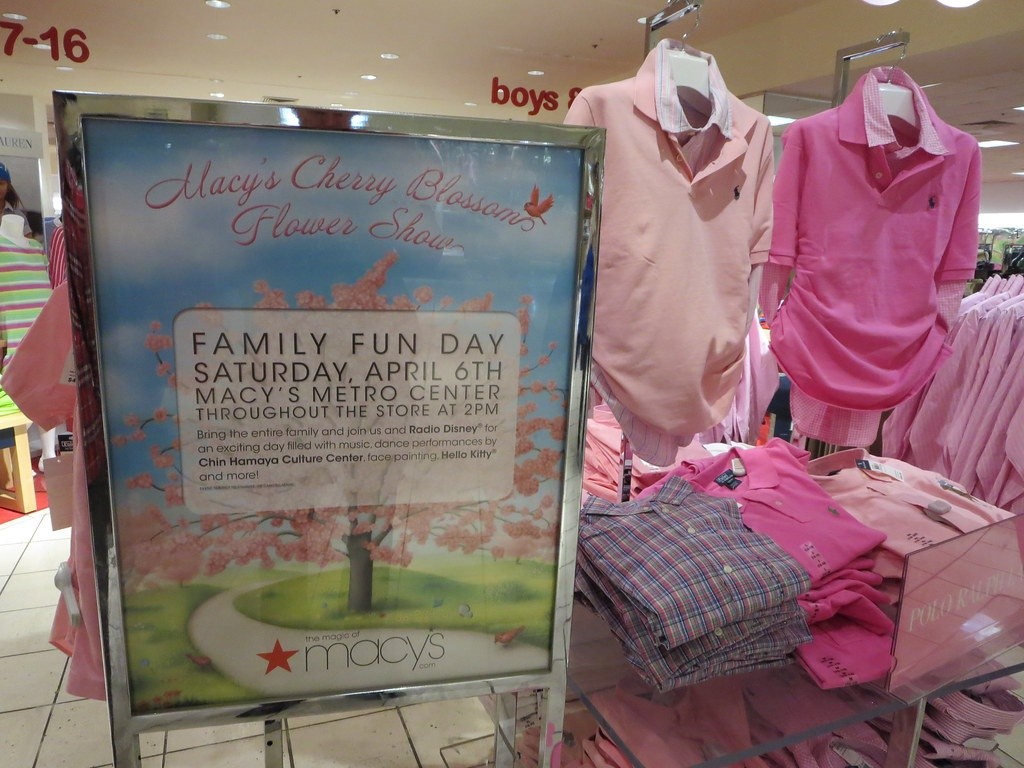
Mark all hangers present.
[783,24,986,158]
[575,0,781,137]
[955,229,1024,333]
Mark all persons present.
[0,161,34,239]
[0,214,76,477]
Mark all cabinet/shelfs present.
[493,569,1024,768]
